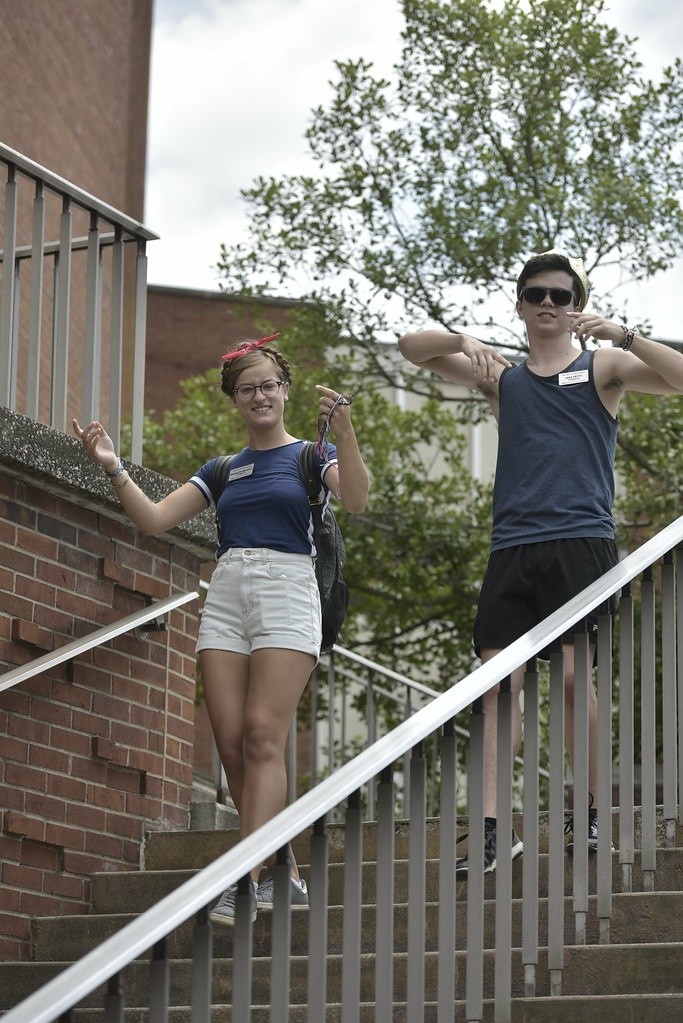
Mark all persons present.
[73,341,370,926]
[398,254,683,882]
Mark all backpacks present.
[298,442,350,656]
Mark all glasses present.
[231,381,288,402]
[519,286,577,306]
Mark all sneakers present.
[209,882,258,925]
[456,822,523,877]
[257,875,310,910]
[564,792,615,854]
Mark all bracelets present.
[110,470,129,489]
[105,457,125,478]
[613,325,636,350]
